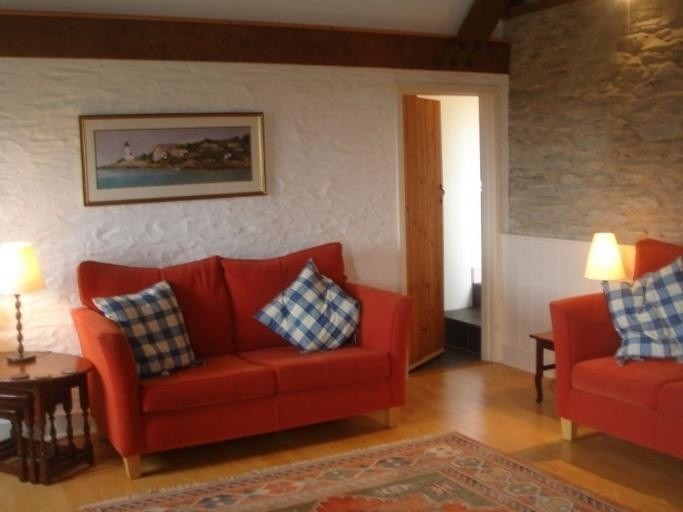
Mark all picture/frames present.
[77,112,268,208]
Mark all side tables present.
[2,350,96,485]
[527,331,556,403]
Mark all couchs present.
[70,241,415,480]
[548,240,683,459]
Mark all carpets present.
[73,428,637,512]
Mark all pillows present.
[600,256,683,369]
[90,255,361,379]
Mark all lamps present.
[585,231,626,292]
[0,240,48,364]
